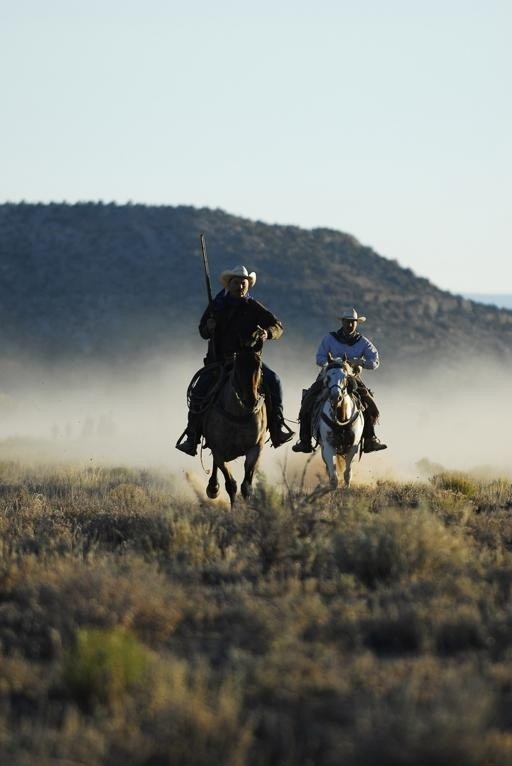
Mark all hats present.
[337,309,366,323]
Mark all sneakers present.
[176,440,196,456]
[272,431,294,448]
[364,440,386,453]
[292,442,312,453]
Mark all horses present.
[316,352,366,492]
[201,335,269,513]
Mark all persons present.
[291,308,386,455]
[177,265,296,456]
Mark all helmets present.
[219,266,256,289]
